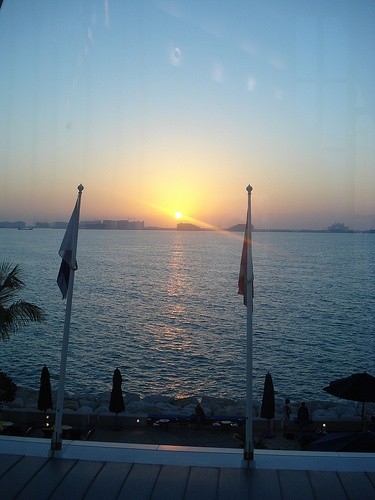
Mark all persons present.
[296,402,310,421]
[195,403,204,417]
[281,399,291,438]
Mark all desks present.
[221,421,232,433]
[159,418,170,433]
[0,420,15,435]
[297,423,328,450]
[62,424,73,440]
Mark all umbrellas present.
[261,372,275,424]
[322,371,375,432]
[37,365,53,413]
[108,367,125,417]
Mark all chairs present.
[24,424,34,436]
[230,422,239,433]
[86,428,96,441]
[212,422,221,430]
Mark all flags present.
[238,209,255,312]
[56,195,82,300]
